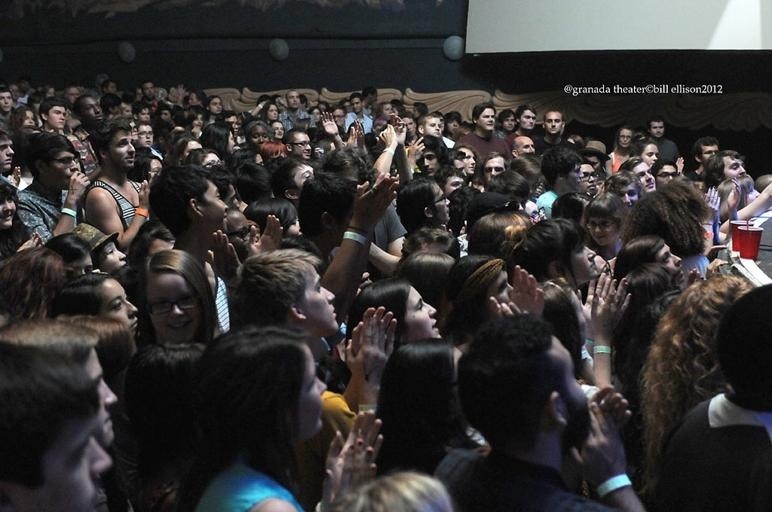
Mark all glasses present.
[532,207,545,218]
[150,295,198,314]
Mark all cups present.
[737,226,763,259]
[729,220,754,251]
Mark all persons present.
[0,80,772,511]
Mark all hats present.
[73,223,118,253]
[577,140,611,160]
